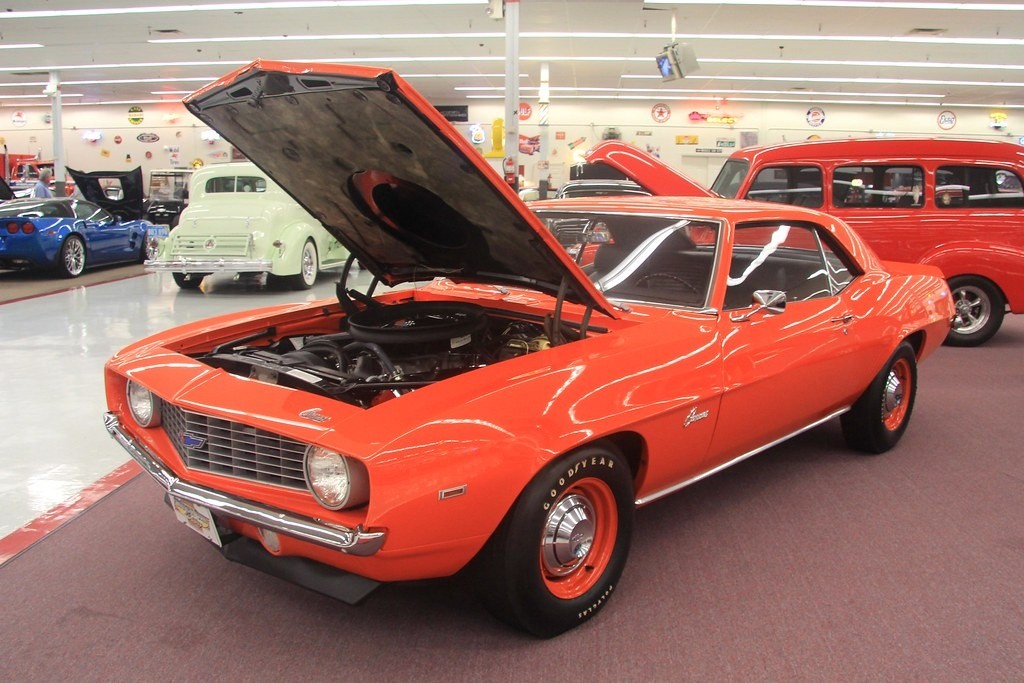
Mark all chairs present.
[588,243,648,289]
[733,254,823,301]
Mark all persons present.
[69,171,86,201]
[30,168,53,198]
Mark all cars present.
[102,57,954,639]
[0,160,352,289]
[582,137,1024,347]
